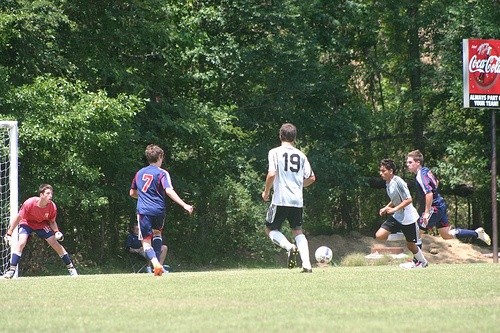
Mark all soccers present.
[315,246,333,264]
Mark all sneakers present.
[300,267,312,273]
[287,244,298,269]
[4,268,15,279]
[475,227,491,245]
[68,268,78,278]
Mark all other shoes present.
[411,256,428,268]
[154,265,163,276]
[147,266,152,273]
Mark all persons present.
[130,144,193,275]
[375,159,428,268]
[406,150,491,245]
[262,124,316,273]
[126,223,167,275]
[3,184,79,278]
